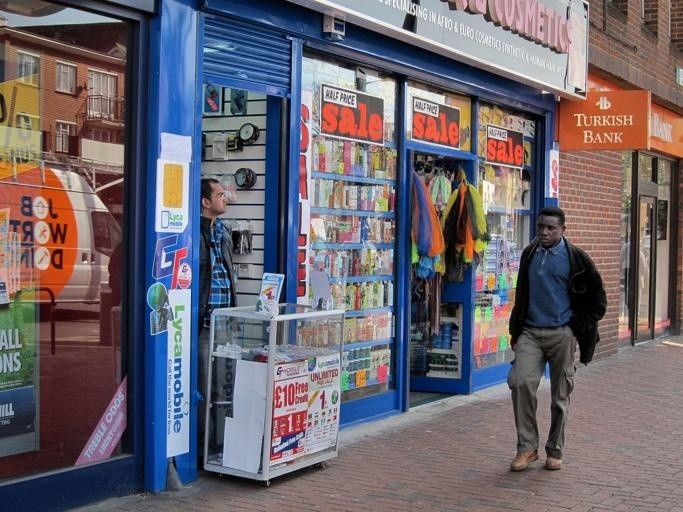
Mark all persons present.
[620,232,630,307]
[198,178,238,441]
[637,250,646,321]
[506,206,607,472]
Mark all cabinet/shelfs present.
[309,135,397,354]
[203,303,345,481]
[473,208,531,357]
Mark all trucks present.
[0,160,126,306]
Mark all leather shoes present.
[545,454,564,470]
[511,448,540,472]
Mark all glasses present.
[206,192,226,199]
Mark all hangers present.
[418,162,441,177]
